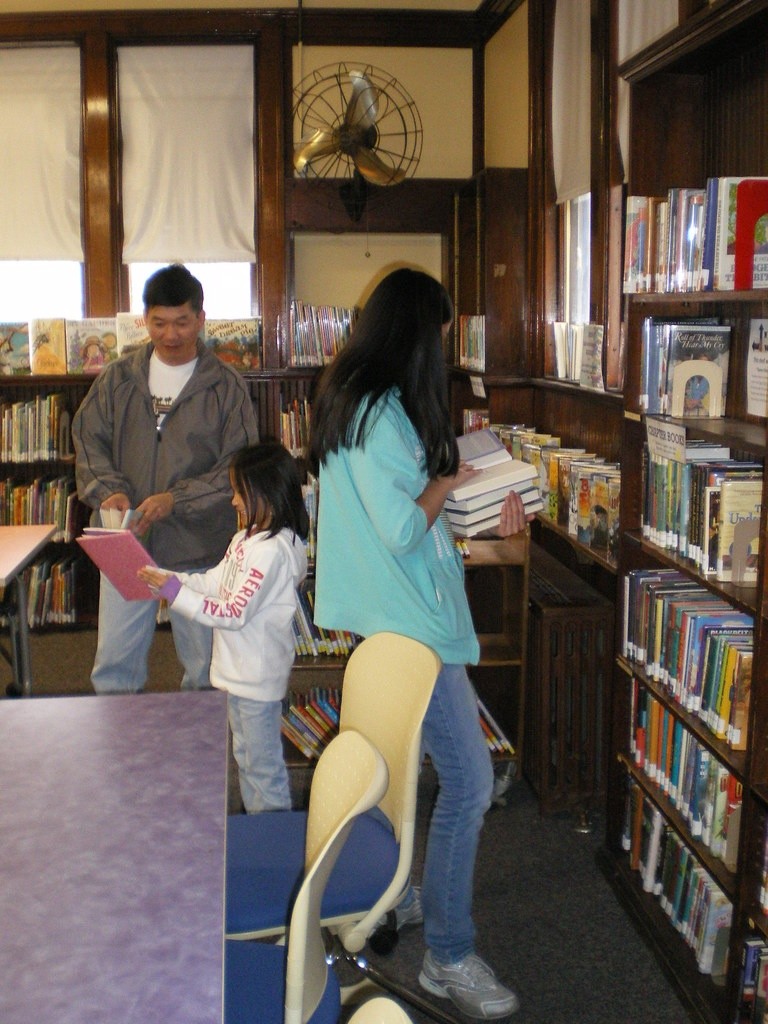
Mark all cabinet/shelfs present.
[0,288,768,1024]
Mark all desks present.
[0,689,229,1024]
[0,524,58,700]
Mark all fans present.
[283,62,423,223]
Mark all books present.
[489,424,621,570]
[157,599,170,624]
[75,508,160,602]
[427,428,544,538]
[629,678,768,920]
[0,392,67,464]
[620,768,768,1024]
[621,567,755,751]
[622,176,768,293]
[0,550,88,629]
[459,315,485,373]
[463,409,489,438]
[0,473,79,544]
[289,300,361,368]
[469,679,515,754]
[553,322,605,391]
[289,575,364,657]
[279,392,310,460]
[641,440,763,588]
[300,470,319,560]
[0,312,262,375]
[281,685,342,763]
[639,314,732,421]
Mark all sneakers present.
[357,885,425,929]
[418,948,518,1020]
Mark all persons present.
[311,269,535,1020]
[137,442,310,814]
[72,262,260,696]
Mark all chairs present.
[224,729,389,1024]
[222,633,441,940]
